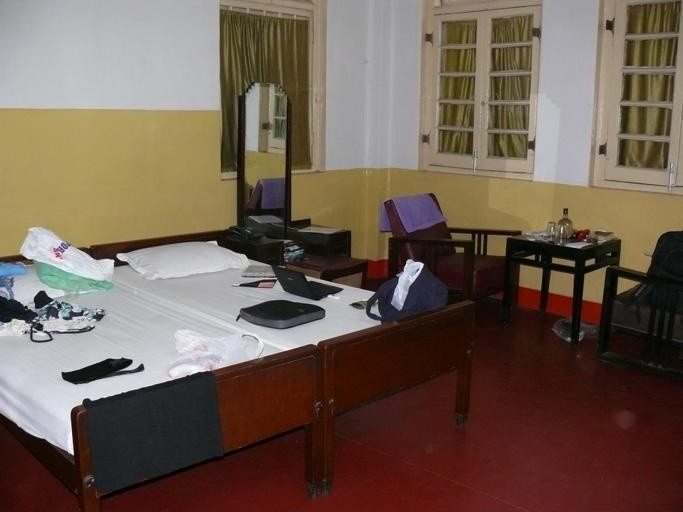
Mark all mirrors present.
[236,84,293,230]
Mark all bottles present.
[558,208,573,240]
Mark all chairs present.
[385,194,516,335]
[599,231,683,379]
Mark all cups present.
[558,224,567,245]
[547,222,557,241]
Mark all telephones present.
[224,224,265,244]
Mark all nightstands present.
[242,233,376,290]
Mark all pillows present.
[8,265,104,302]
[116,241,252,277]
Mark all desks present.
[502,232,621,350]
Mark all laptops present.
[271,264,343,301]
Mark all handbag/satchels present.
[366,259,447,324]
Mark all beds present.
[90,228,479,495]
[0,247,323,509]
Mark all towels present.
[247,176,285,209]
[379,194,446,233]
